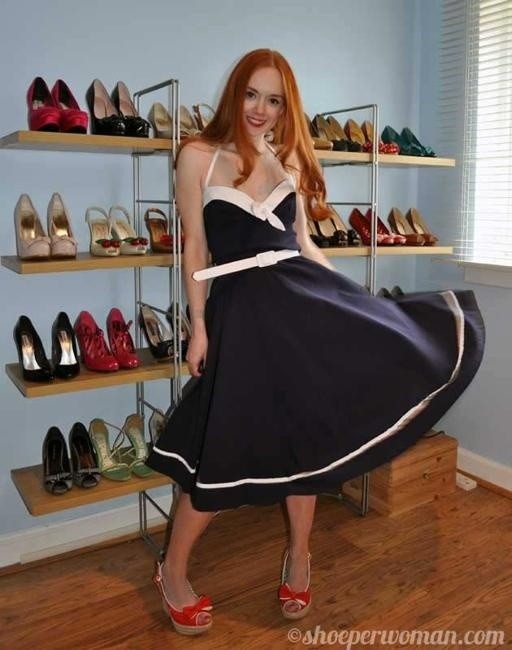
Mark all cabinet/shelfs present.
[0,78,455,569]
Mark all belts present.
[191,248,301,282]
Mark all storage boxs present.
[343,429,459,516]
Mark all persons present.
[146,49,484,637]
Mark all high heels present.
[307,211,330,248]
[73,311,120,372]
[144,208,178,254]
[138,304,179,362]
[86,78,126,136]
[361,120,399,155]
[381,125,425,157]
[193,103,216,131]
[27,77,62,133]
[277,547,313,620]
[147,102,192,140]
[349,208,394,246]
[51,311,80,380]
[172,208,185,254]
[42,426,74,495]
[13,194,52,261]
[303,112,334,151]
[364,207,407,246]
[149,410,169,447]
[69,422,102,488]
[111,80,151,137]
[109,205,148,255]
[401,128,437,157]
[106,308,139,369]
[85,207,121,257]
[328,204,362,247]
[152,560,213,636]
[13,315,54,382]
[113,414,154,478]
[47,193,78,259]
[51,79,88,134]
[388,207,426,246]
[344,119,387,154]
[312,114,346,151]
[313,217,349,248]
[166,302,192,362]
[326,116,363,152]
[88,419,131,481]
[175,104,202,137]
[406,207,439,246]
